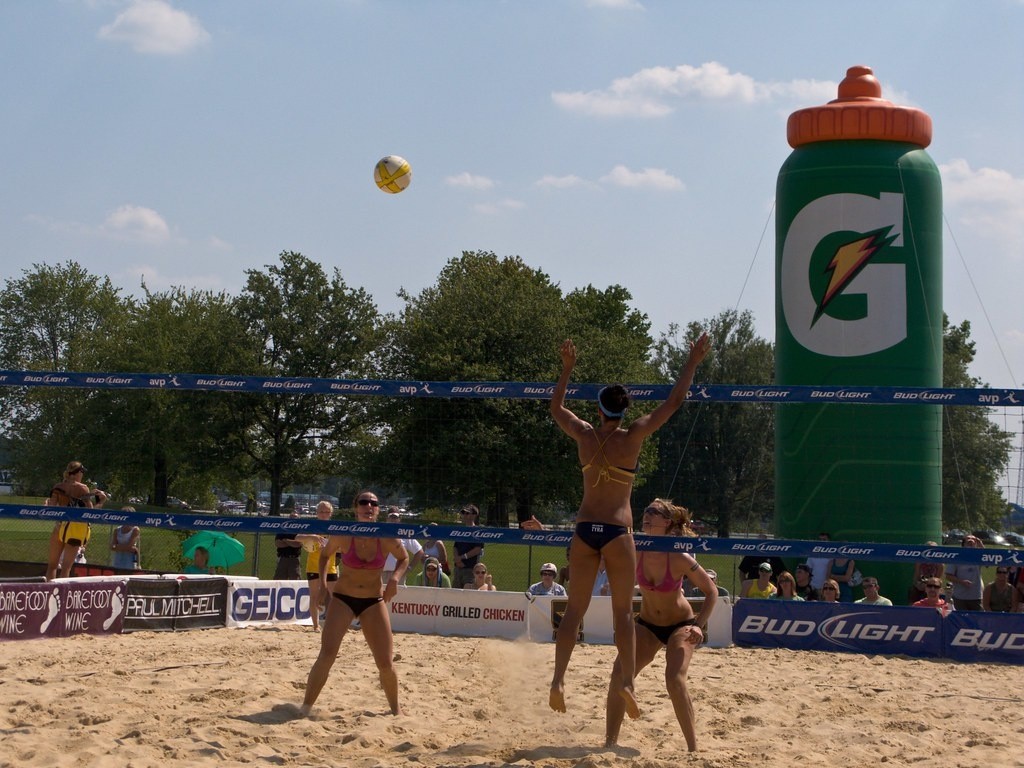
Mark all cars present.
[167,496,192,510]
[218,500,339,515]
[379,505,420,519]
[942,530,1024,548]
[685,520,714,536]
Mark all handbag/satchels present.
[847,567,864,587]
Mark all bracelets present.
[464,553,468,560]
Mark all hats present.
[69,464,88,475]
[540,563,557,573]
[759,562,771,571]
[461,504,478,514]
[796,564,813,577]
[705,569,717,578]
[426,558,438,568]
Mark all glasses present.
[358,500,379,506]
[997,571,1008,574]
[863,585,873,589]
[780,579,790,585]
[927,584,939,589]
[543,573,554,576]
[760,569,769,573]
[475,571,485,574]
[462,512,472,515]
[643,507,658,516]
[427,568,436,571]
[823,587,836,591]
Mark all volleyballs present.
[373,155,412,194]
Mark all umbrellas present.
[180,529,246,567]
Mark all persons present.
[558,546,729,598]
[382,507,423,585]
[185,547,213,574]
[295,501,339,633]
[945,534,990,611]
[421,522,451,577]
[1007,548,1024,612]
[525,563,567,604]
[273,510,303,580]
[45,461,106,580]
[983,565,1018,612]
[549,332,711,719]
[913,541,944,603]
[464,563,496,591]
[416,557,451,588]
[604,498,719,754]
[854,577,893,606]
[109,505,140,570]
[452,504,485,589]
[737,533,855,603]
[912,578,956,611]
[300,492,409,718]
